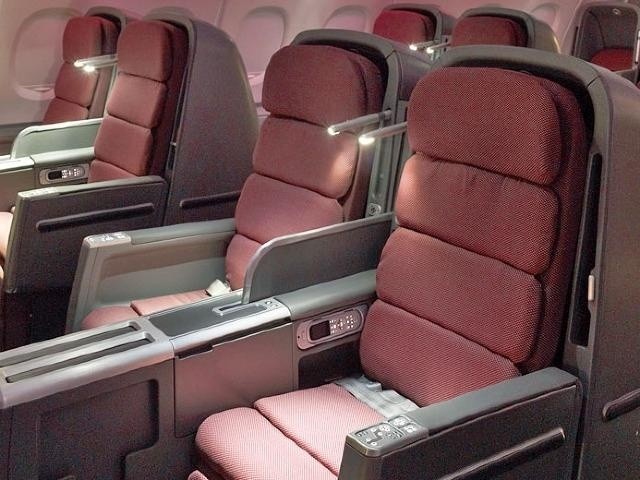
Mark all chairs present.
[0,3,640,476]
